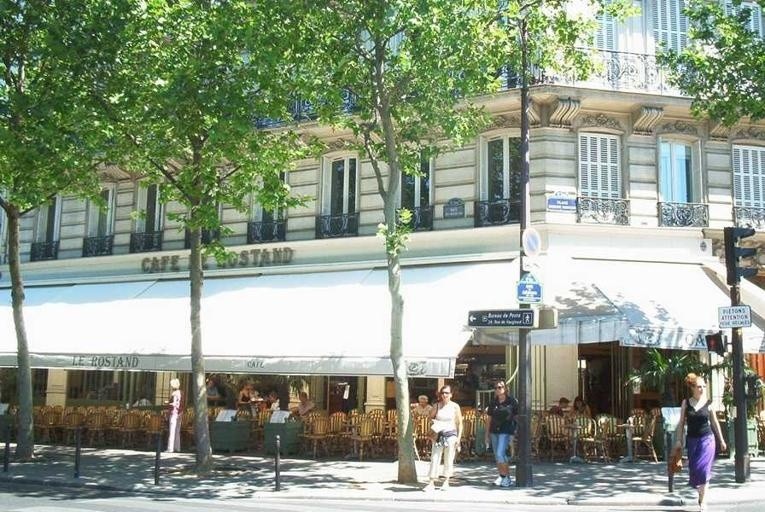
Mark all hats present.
[170,378,180,388]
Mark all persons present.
[551,397,570,417]
[238,381,253,411]
[5,403,19,443]
[413,395,434,416]
[422,385,463,492]
[132,397,154,407]
[485,378,519,487]
[677,373,728,511]
[205,379,220,407]
[569,395,592,419]
[162,378,183,452]
[654,389,676,410]
[267,391,281,409]
[295,390,316,418]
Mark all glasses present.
[441,392,450,394]
[697,385,706,389]
[495,386,502,390]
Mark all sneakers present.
[440,481,449,491]
[164,449,181,453]
[423,484,435,492]
[492,476,504,486]
[501,475,514,487]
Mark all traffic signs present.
[465,308,537,334]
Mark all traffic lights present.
[706,330,728,357]
[724,227,758,286]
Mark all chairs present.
[0,401,666,465]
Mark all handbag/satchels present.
[667,448,683,474]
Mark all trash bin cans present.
[263,410,303,456]
[211,409,251,454]
